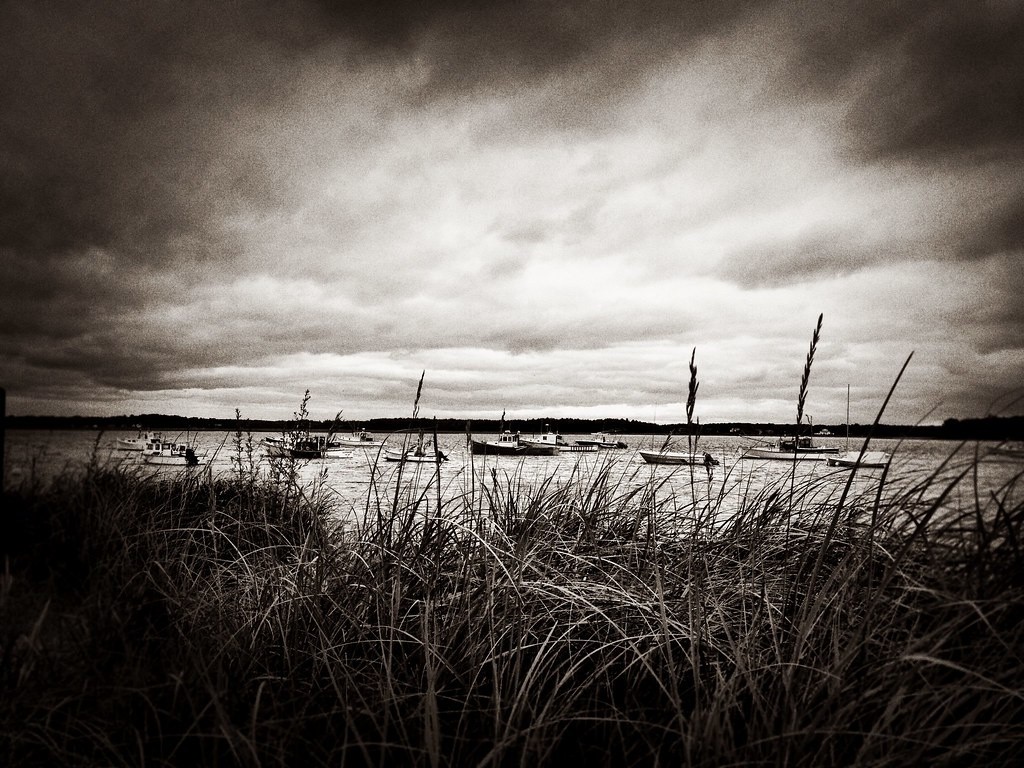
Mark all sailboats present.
[826,384,892,467]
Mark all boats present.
[471,424,598,455]
[985,441,1023,456]
[739,436,840,459]
[264,428,387,458]
[639,450,719,465]
[575,432,617,448]
[116,430,211,465]
[385,448,437,461]
